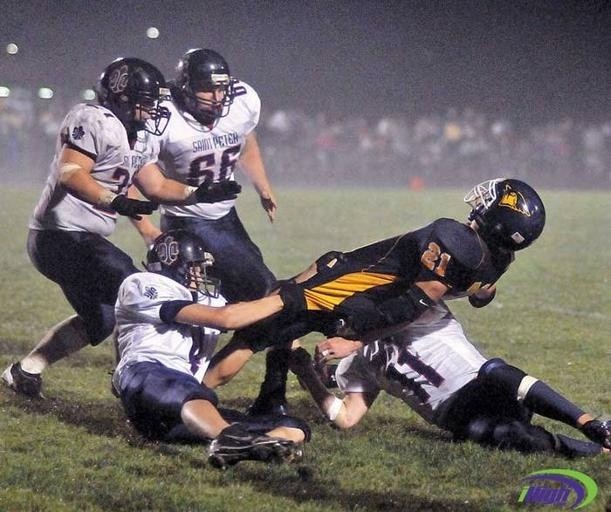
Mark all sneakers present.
[248,381,289,415]
[2,362,47,405]
[209,423,303,470]
[584,416,611,447]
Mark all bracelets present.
[96,190,118,212]
[182,185,198,206]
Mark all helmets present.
[143,229,224,300]
[97,55,171,139]
[173,46,235,124]
[466,170,549,255]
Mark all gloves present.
[194,174,243,201]
[271,282,308,313]
[108,193,160,222]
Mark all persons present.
[110,228,350,471]
[202,178,546,417]
[291,300,611,460]
[1,56,242,405]
[125,48,279,305]
